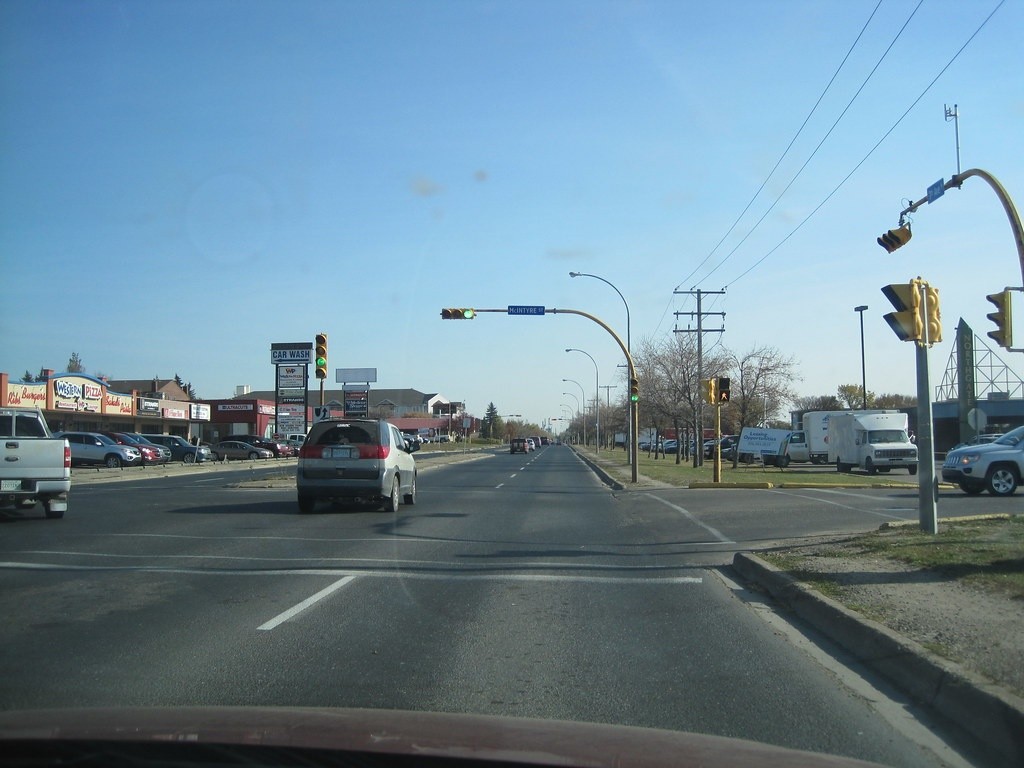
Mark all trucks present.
[777,409,918,475]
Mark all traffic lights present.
[877,227,911,254]
[986,291,1013,347]
[880,284,914,340]
[630,379,639,403]
[716,378,730,402]
[699,379,711,402]
[315,334,328,379]
[441,308,474,319]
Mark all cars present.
[941,426,1024,496]
[209,434,307,461]
[641,435,738,459]
[402,434,449,444]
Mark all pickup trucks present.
[0,406,72,518]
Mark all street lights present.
[560,272,632,464]
[854,306,870,409]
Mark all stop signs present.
[274,434,279,439]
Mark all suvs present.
[510,436,549,454]
[296,417,420,513]
[53,431,211,468]
